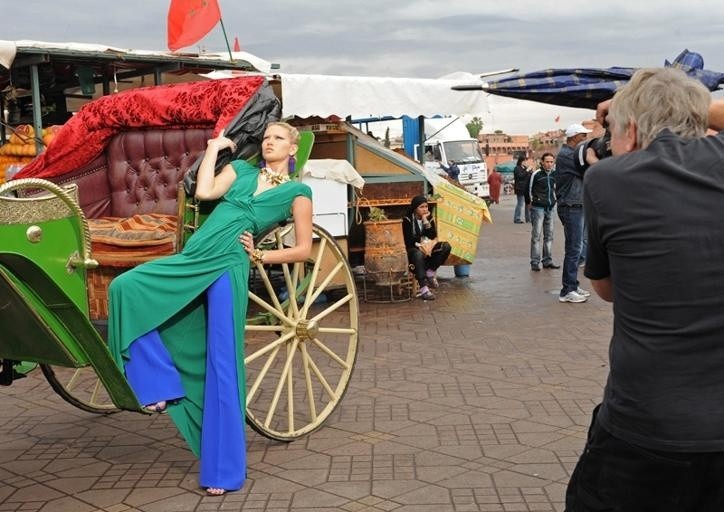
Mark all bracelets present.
[251,248,265,266]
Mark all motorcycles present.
[504,168,536,194]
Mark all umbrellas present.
[451,50,724,111]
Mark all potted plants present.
[363,205,408,288]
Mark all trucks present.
[405,117,496,205]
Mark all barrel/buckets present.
[453,265,470,277]
[362,219,410,284]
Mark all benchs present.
[14,125,218,322]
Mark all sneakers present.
[558,286,591,303]
[513,218,524,223]
[419,285,436,302]
[424,266,440,288]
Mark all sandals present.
[145,399,179,412]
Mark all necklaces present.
[260,166,292,186]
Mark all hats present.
[565,122,595,139]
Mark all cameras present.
[574,110,614,173]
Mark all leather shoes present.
[543,262,561,269]
[530,263,541,271]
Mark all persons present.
[437,160,460,183]
[107,121,314,495]
[403,195,451,301]
[487,168,503,204]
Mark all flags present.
[167,0,221,53]
